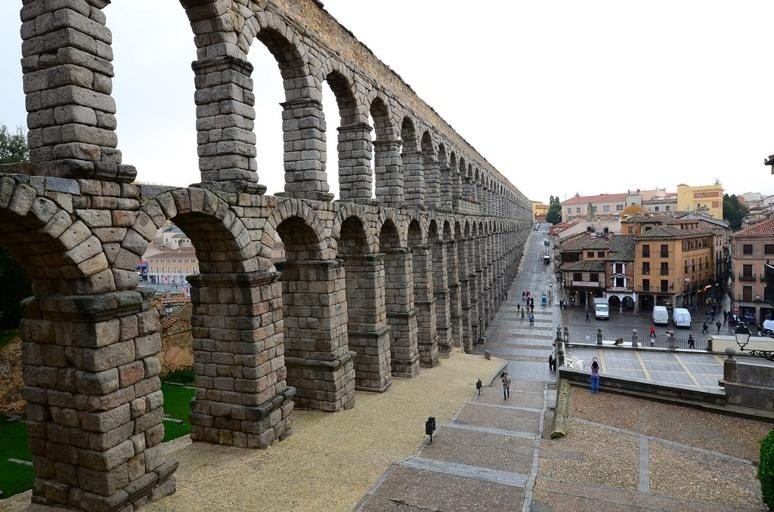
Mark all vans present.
[652,306,691,329]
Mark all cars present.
[762,319,774,334]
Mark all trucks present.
[593,298,610,320]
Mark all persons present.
[688,334,694,349]
[688,303,728,334]
[585,310,591,322]
[501,372,512,400]
[590,361,601,393]
[649,321,657,337]
[517,289,535,325]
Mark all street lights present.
[733,322,774,362]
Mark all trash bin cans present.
[485,350,491,360]
[426,417,435,443]
